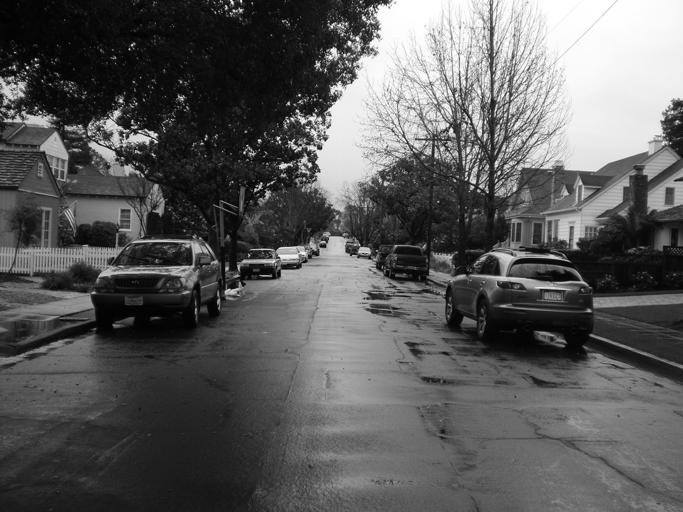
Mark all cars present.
[376,245,392,268]
[445,246,595,357]
[240,248,281,279]
[343,233,349,238]
[308,232,331,258]
[345,239,371,259]
[276,246,308,268]
[92,238,226,326]
[383,245,428,280]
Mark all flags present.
[62,200,80,238]
